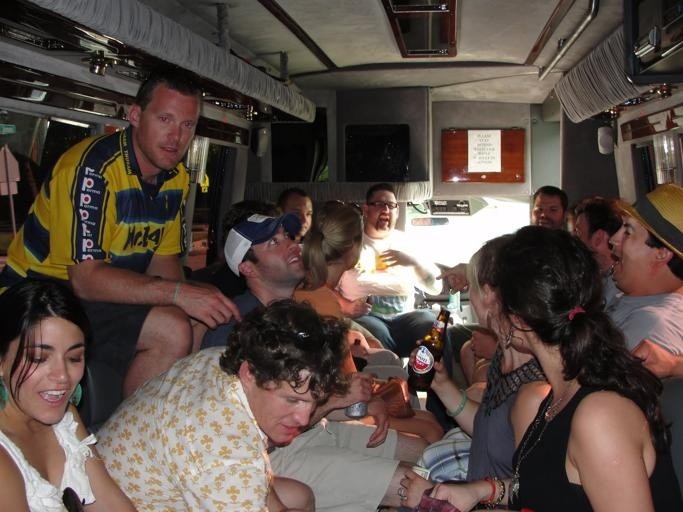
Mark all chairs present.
[659,379,682,497]
[82,358,132,442]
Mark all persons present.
[206,183,444,511]
[92,298,354,512]
[398,182,683,511]
[0,273,135,512]
[0,69,244,404]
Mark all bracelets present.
[172,279,182,306]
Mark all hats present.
[615,182,682,258]
[223,211,301,277]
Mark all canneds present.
[345,400,367,418]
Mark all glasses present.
[295,330,326,352]
[367,200,399,210]
[327,199,364,219]
[62,487,86,511]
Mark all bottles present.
[408,308,450,393]
[350,339,366,373]
